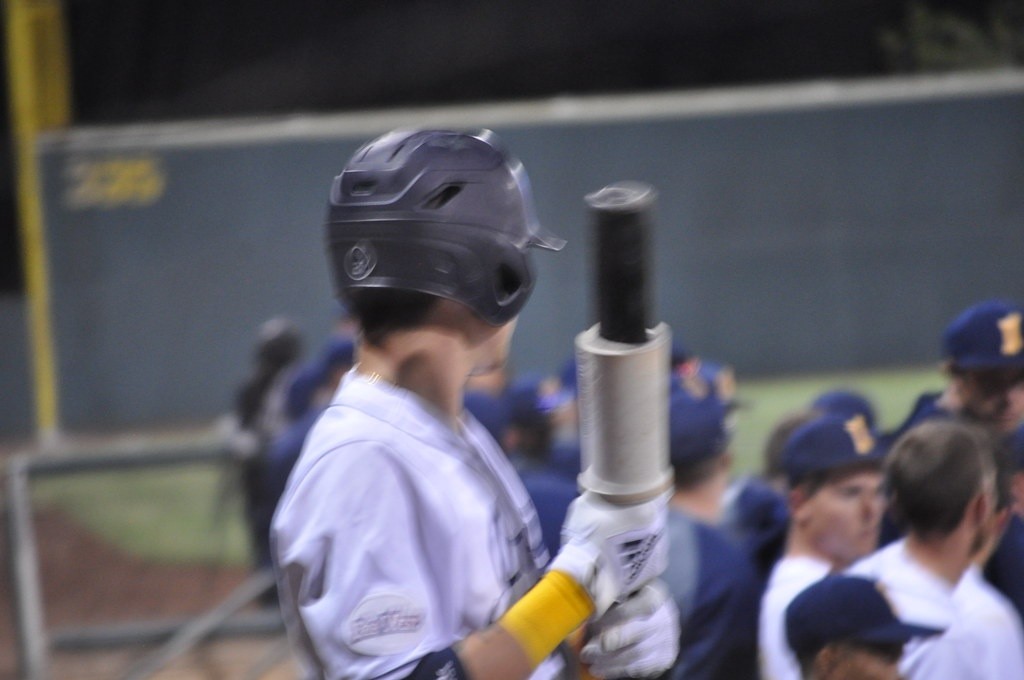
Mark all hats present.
[780,416,895,484]
[281,335,578,447]
[785,575,944,658]
[814,392,874,427]
[669,392,734,460]
[946,300,1024,371]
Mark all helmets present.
[327,128,567,326]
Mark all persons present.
[265,130,680,678]
[245,310,1023,680]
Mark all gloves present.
[580,577,682,680]
[545,486,676,621]
